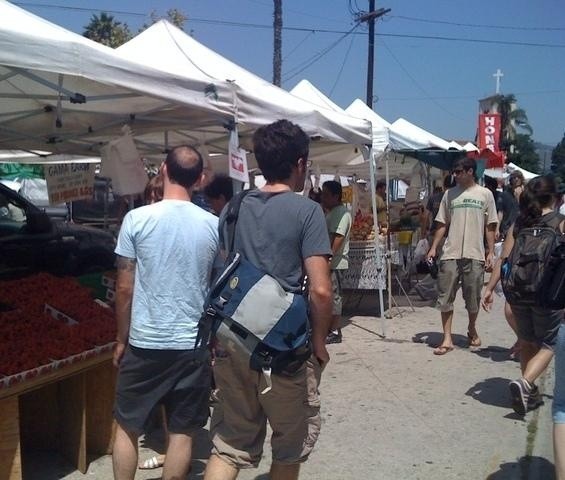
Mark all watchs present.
[328,256,333,261]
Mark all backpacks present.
[505,214,565,310]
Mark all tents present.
[112,17,384,320]
[0,2,239,158]
[289,80,541,185]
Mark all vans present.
[0,180,118,278]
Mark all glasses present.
[305,159,312,170]
[449,167,465,175]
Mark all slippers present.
[432,342,454,355]
[467,331,482,346]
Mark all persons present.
[482,174,565,416]
[425,175,457,257]
[187,158,213,215]
[203,176,245,403]
[494,181,504,211]
[375,181,395,229]
[550,183,565,215]
[206,119,334,480]
[427,156,499,356]
[314,180,352,343]
[138,173,192,471]
[494,170,528,297]
[111,145,222,479]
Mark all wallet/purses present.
[427,256,438,279]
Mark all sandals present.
[140,457,165,469]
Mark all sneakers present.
[508,377,528,418]
[324,333,341,343]
[528,387,544,411]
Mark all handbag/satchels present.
[193,187,315,395]
